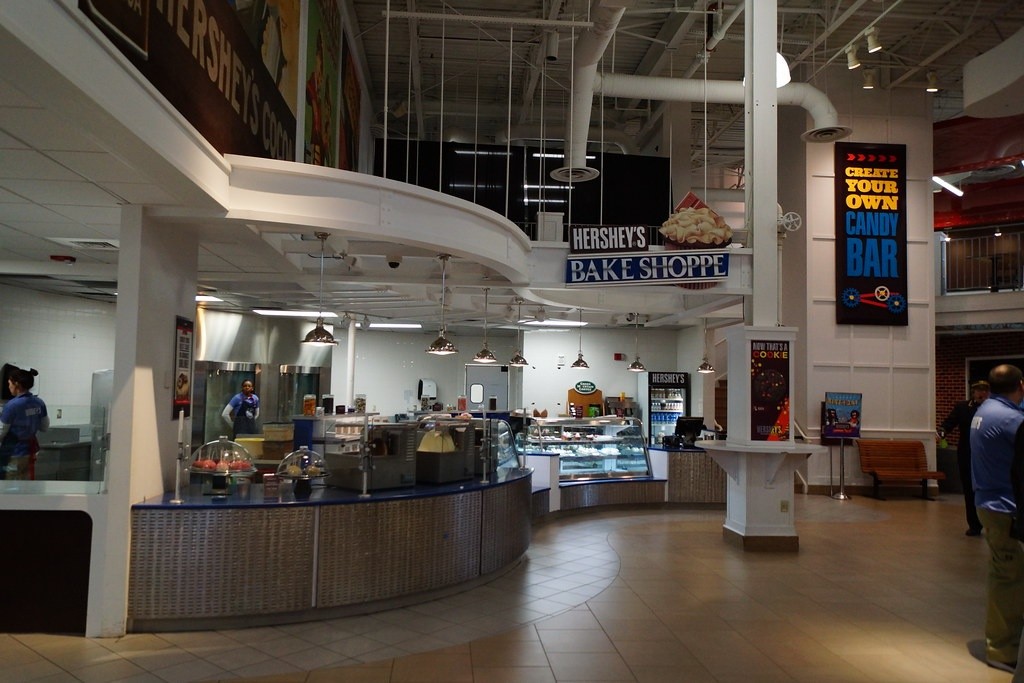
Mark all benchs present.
[857,438,947,500]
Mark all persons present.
[936,377,992,536]
[0,368,49,480]
[848,410,859,427]
[969,364,1024,673]
[826,409,838,425]
[221,380,260,438]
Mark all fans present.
[516,307,564,325]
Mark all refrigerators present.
[638,371,691,448]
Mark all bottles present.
[355,393,367,414]
[651,435,655,445]
[322,394,334,415]
[458,394,467,411]
[569,402,583,418]
[651,388,683,425]
[413,397,456,412]
[589,403,600,416]
[303,394,316,417]
[489,394,497,411]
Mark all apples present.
[193,458,252,472]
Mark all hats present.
[968,375,993,389]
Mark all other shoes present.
[986,654,1019,672]
[966,527,982,536]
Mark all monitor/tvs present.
[674,416,703,449]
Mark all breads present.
[287,465,320,476]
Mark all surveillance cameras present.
[386,255,402,268]
[625,313,633,322]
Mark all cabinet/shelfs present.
[527,418,654,480]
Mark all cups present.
[347,404,355,415]
[336,405,344,415]
[619,392,625,402]
[316,407,325,417]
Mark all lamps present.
[472,287,497,363]
[505,306,518,322]
[861,70,875,89]
[340,311,352,328]
[695,317,715,374]
[994,227,1002,237]
[845,44,860,70]
[626,312,646,373]
[934,173,964,198]
[863,26,883,53]
[570,308,589,369]
[508,297,528,367]
[740,51,791,88]
[440,286,453,307]
[545,31,560,61]
[300,232,340,346]
[426,254,459,356]
[925,69,939,94]
[360,315,371,331]
